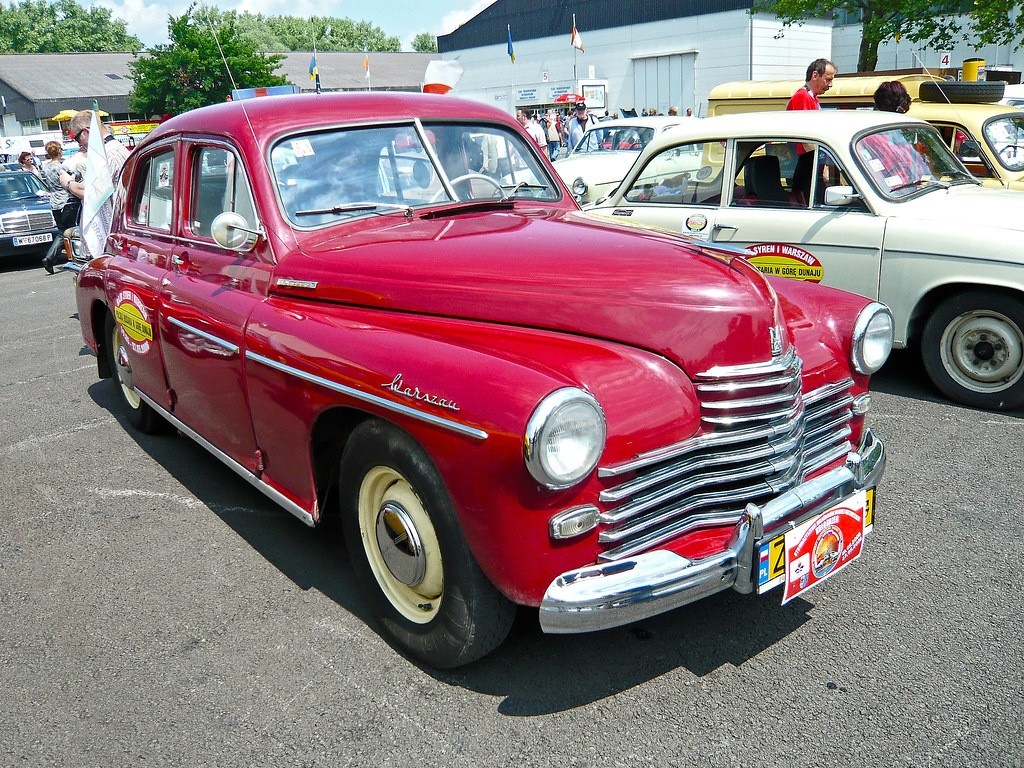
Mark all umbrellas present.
[51,109,79,122]
[84,109,109,117]
[554,93,585,112]
[66,179,77,191]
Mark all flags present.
[507,27,515,64]
[893,13,901,43]
[571,26,585,53]
[424,60,463,95]
[309,49,317,81]
[361,55,370,79]
[81,111,113,259]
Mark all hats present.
[576,102,586,109]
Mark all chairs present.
[744,155,790,207]
[792,149,827,205]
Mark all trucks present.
[700,74,1024,194]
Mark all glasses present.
[24,158,33,161]
[74,126,90,142]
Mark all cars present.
[579,109,1024,419]
[497,116,707,210]
[74,89,899,672]
[0,146,79,262]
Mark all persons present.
[602,110,619,122]
[786,58,837,182]
[483,103,604,179]
[0,148,42,184]
[649,107,695,117]
[60,111,130,198]
[857,81,942,189]
[42,140,87,274]
[225,92,233,102]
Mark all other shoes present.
[42,259,54,274]
[550,158,555,162]
